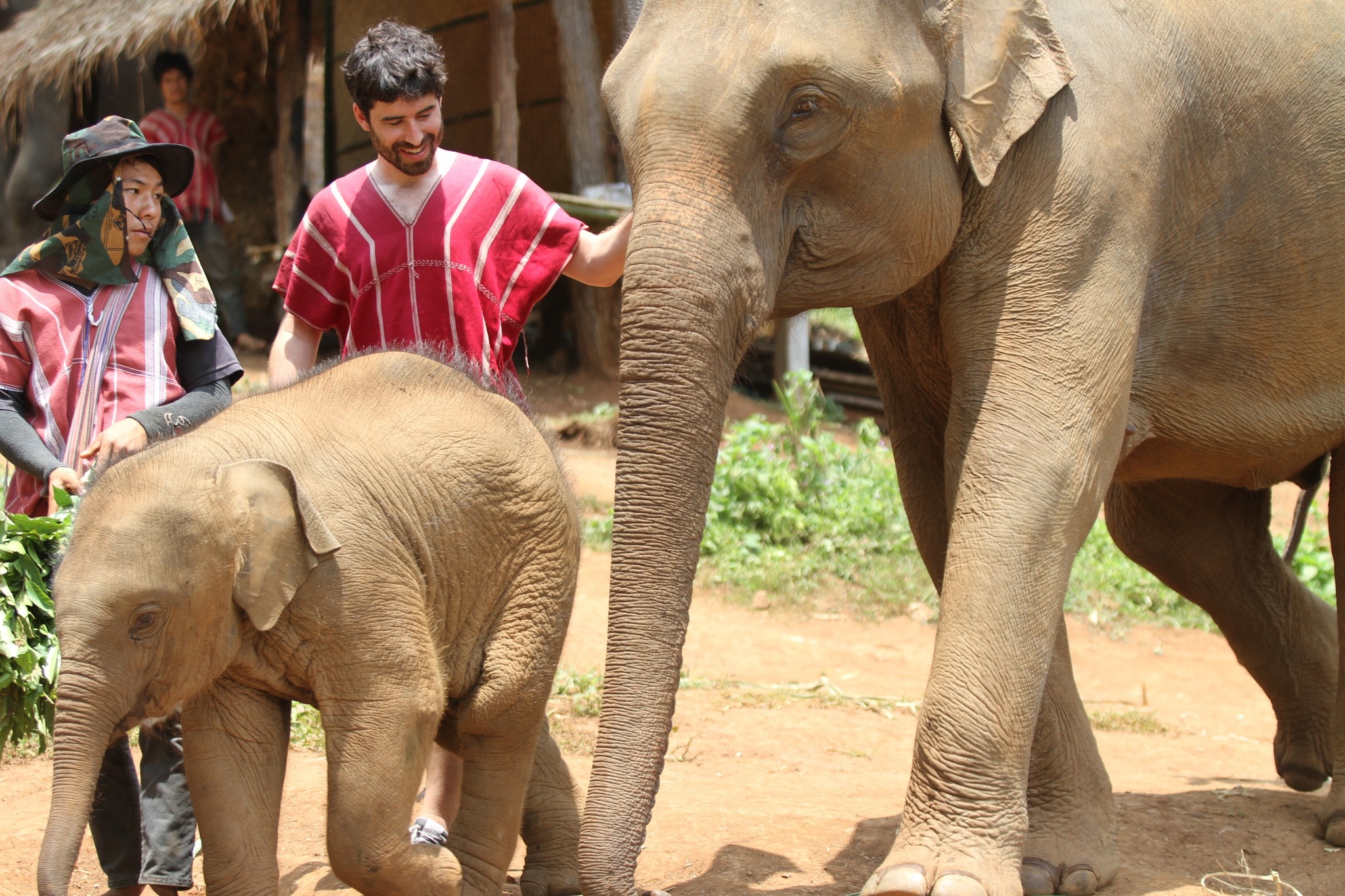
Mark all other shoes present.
[404,817,451,850]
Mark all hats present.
[33,114,194,222]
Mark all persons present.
[139,51,268,354]
[0,115,246,896]
[267,17,632,848]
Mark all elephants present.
[576,3,1344,896]
[37,339,586,896]
